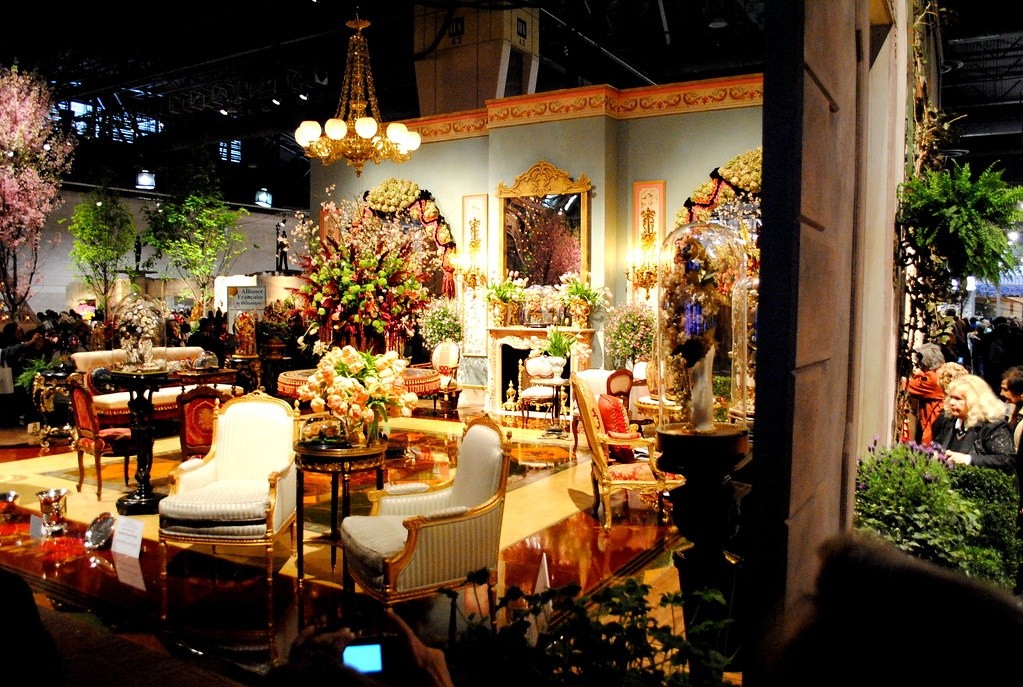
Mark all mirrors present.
[496,158,592,286]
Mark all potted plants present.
[13,350,76,440]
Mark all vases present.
[347,419,367,448]
[549,356,566,382]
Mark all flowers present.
[274,178,464,353]
[297,337,419,449]
[604,301,659,364]
[485,269,614,360]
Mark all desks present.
[655,422,750,527]
[294,438,388,594]
[280,365,441,400]
[635,395,722,425]
[174,369,238,399]
[225,354,261,391]
[107,373,170,516]
[30,365,79,450]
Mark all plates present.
[84,512,115,549]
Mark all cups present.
[0,491,20,521]
[38,488,69,534]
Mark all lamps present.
[624,207,658,301]
[218,97,228,117]
[281,213,287,227]
[298,85,310,101]
[295,20,421,181]
[254,186,274,209]
[448,218,488,300]
[272,93,282,106]
[132,129,157,191]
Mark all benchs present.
[70,347,243,427]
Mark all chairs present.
[64,337,685,669]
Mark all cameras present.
[340,633,400,678]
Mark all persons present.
[943,309,1023,395]
[661,236,731,435]
[167,311,234,368]
[902,343,1023,471]
[254,610,453,687]
[0,309,102,426]
